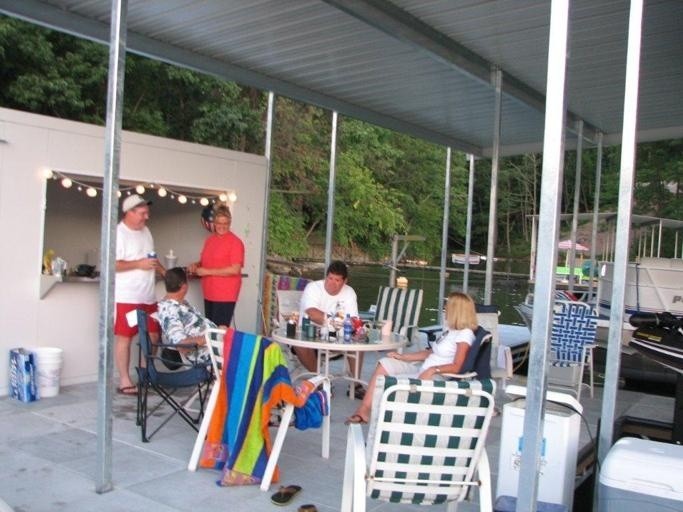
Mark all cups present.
[164,254,179,270]
[147,251,158,266]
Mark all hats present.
[122,194,152,213]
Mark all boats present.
[510,210,682,387]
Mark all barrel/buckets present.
[33,345,63,398]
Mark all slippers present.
[345,415,369,425]
[301,505,315,512]
[117,385,138,395]
[271,486,301,505]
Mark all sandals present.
[347,384,366,399]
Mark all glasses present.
[436,331,449,343]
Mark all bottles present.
[281,310,392,344]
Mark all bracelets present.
[434,364,441,375]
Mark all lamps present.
[35,162,237,213]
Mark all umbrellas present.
[556,237,589,266]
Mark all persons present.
[292,258,367,401]
[345,291,478,427]
[185,204,245,330]
[114,193,168,397]
[156,266,228,381]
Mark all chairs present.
[513,285,598,403]
